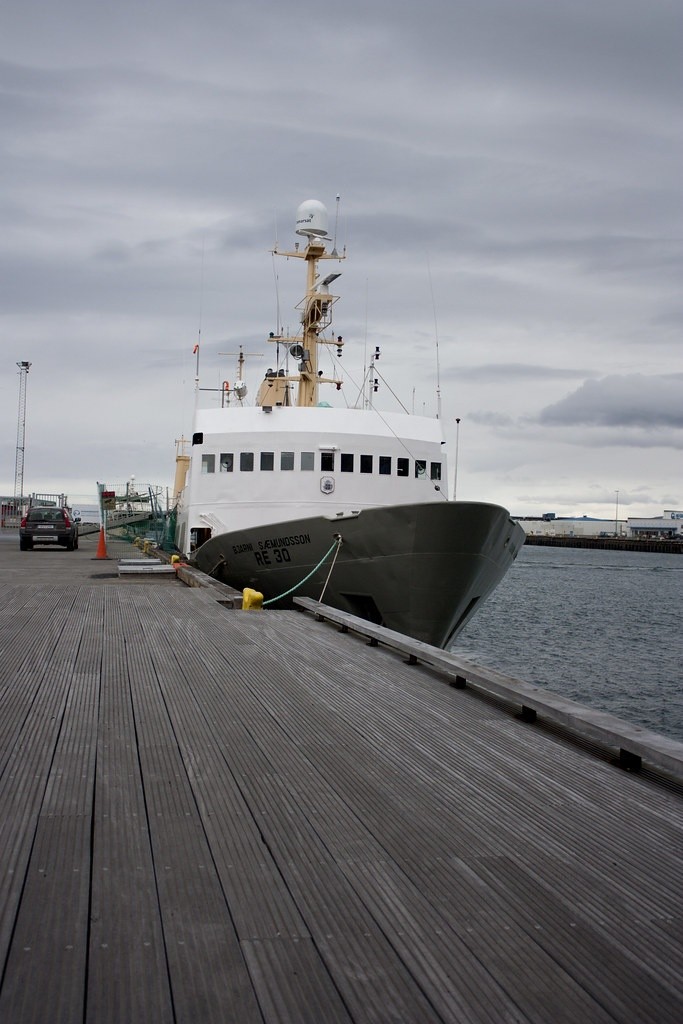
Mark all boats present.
[172,194,526,650]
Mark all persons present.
[265,366,289,406]
[46,515,52,520]
[58,513,62,519]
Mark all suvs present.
[19,506,81,552]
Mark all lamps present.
[276,401,282,406]
[263,405,273,414]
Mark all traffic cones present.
[91,526,112,560]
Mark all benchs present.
[30,513,63,519]
